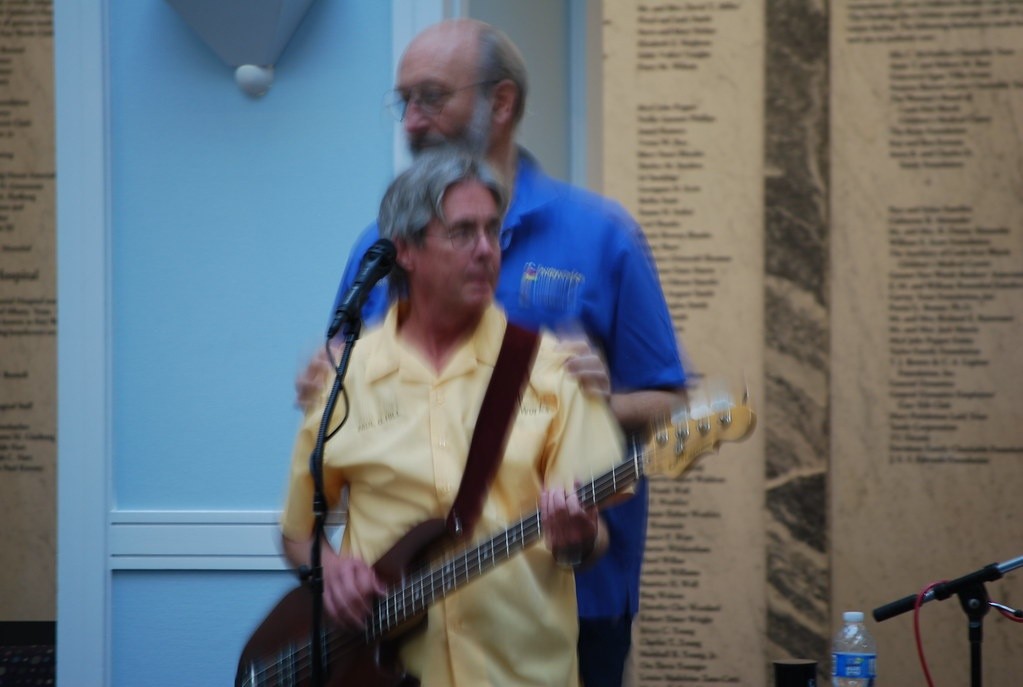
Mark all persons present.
[276,151,633,687]
[297,15,691,687]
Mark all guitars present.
[234,379,758,687]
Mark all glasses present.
[385,79,495,122]
[415,224,506,251]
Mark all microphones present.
[327,238,398,338]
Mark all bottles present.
[830,612,877,686]
